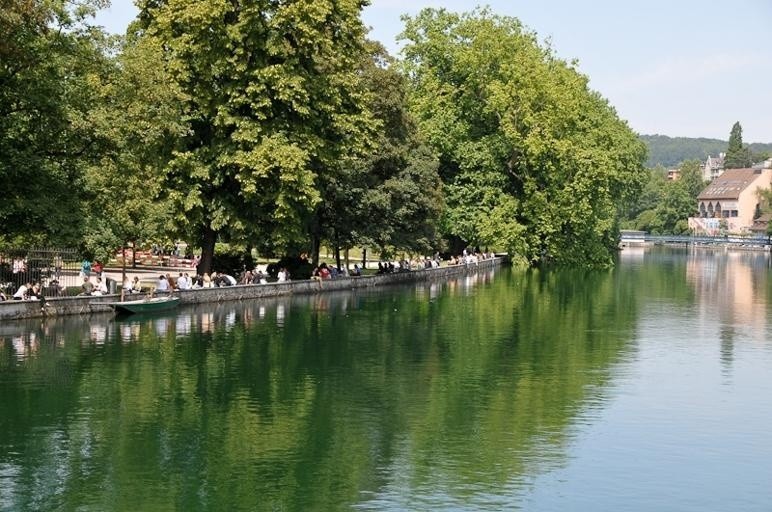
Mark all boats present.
[87,293,181,315]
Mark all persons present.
[0,237,494,314]
[0,300,298,368]
